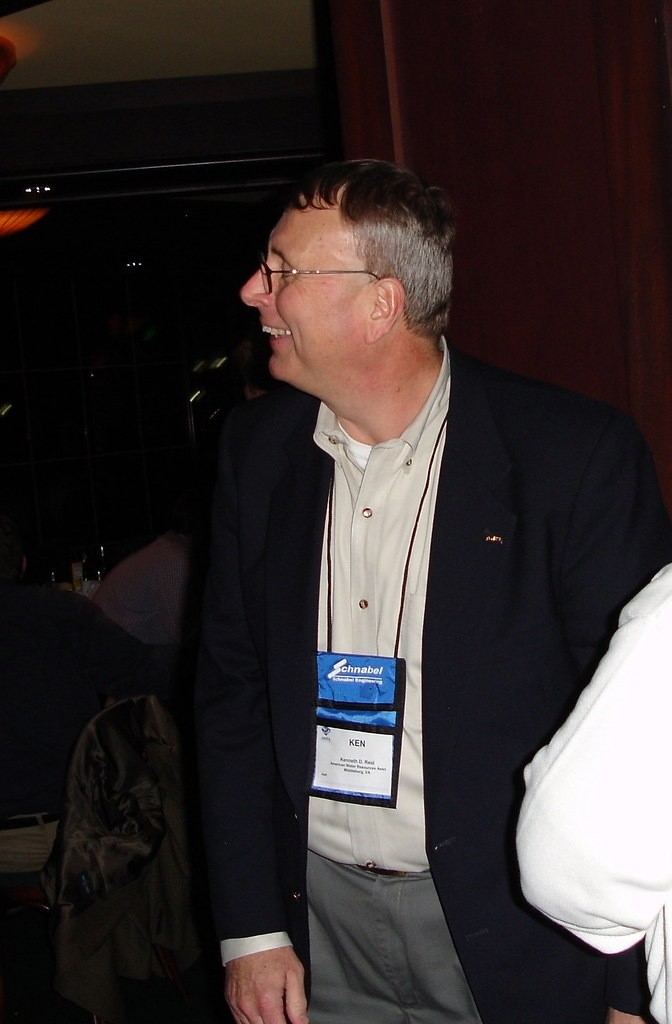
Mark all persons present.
[514,563,672,1024]
[0,299,270,874]
[194,157,672,1024]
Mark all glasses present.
[256,250,383,295]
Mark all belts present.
[1,813,60,831]
